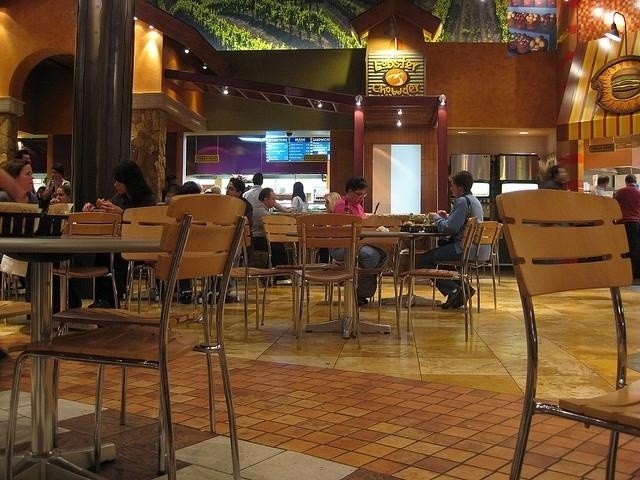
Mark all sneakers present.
[440,284,476,309]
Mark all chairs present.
[358,213,410,337]
[40,203,73,235]
[396,216,478,344]
[1,201,43,236]
[293,216,362,350]
[433,221,504,310]
[230,215,278,341]
[53,212,122,309]
[263,214,304,324]
[123,205,196,322]
[6,194,247,480]
[494,190,640,479]
[398,214,443,308]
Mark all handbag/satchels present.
[437,237,455,247]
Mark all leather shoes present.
[88,302,111,308]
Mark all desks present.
[286,232,439,340]
[0,238,166,480]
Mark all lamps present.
[185,49,189,54]
[605,12,628,56]
[134,17,138,20]
[202,63,208,70]
[149,25,154,29]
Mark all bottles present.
[480,199,490,217]
[423,211,430,225]
[409,213,415,224]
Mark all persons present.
[613,175,640,280]
[421,170,491,310]
[589,175,611,198]
[0,149,73,204]
[163,173,309,304]
[537,165,569,264]
[82,161,157,309]
[320,176,389,305]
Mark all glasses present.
[353,190,369,197]
